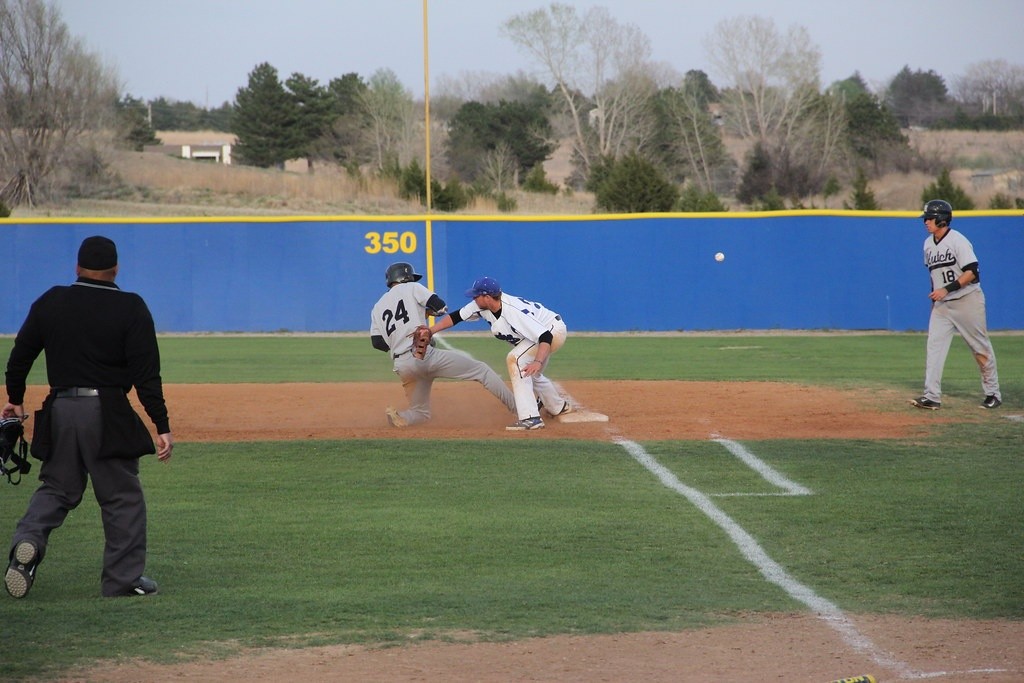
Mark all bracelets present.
[534,360,543,365]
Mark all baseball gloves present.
[411,325,432,362]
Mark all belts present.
[56,387,99,398]
[556,315,562,321]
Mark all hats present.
[77,236,118,270]
[464,275,500,298]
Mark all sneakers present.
[980,396,1002,410]
[127,578,158,597]
[559,402,573,414]
[908,397,940,410]
[3,539,38,599]
[505,417,545,431]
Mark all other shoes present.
[537,397,542,411]
[386,405,406,429]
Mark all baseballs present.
[714,252,724,262]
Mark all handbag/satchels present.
[30,393,56,461]
[97,390,156,460]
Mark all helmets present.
[385,262,423,287]
[918,199,953,227]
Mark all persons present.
[370,263,543,427]
[0,235,173,599]
[907,200,1002,408]
[412,276,571,433]
